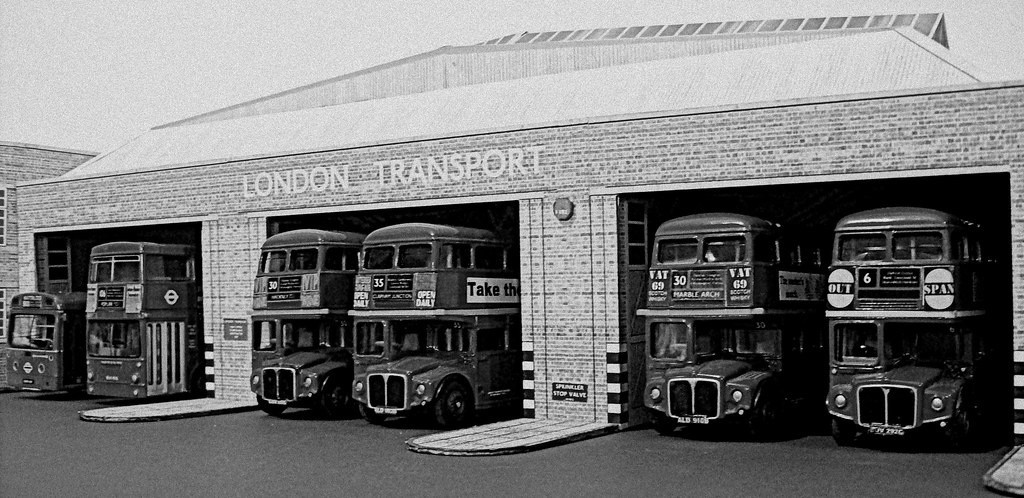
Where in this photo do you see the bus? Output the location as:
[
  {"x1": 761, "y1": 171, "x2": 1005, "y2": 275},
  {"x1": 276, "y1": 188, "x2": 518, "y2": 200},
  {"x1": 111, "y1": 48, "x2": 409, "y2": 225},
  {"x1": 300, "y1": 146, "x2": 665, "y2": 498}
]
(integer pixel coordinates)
[
  {"x1": 347, "y1": 223, "x2": 521, "y2": 430},
  {"x1": 636, "y1": 213, "x2": 833, "y2": 442},
  {"x1": 6, "y1": 292, "x2": 87, "y2": 396},
  {"x1": 85, "y1": 241, "x2": 205, "y2": 405},
  {"x1": 249, "y1": 229, "x2": 367, "y2": 418},
  {"x1": 825, "y1": 207, "x2": 1011, "y2": 452}
]
